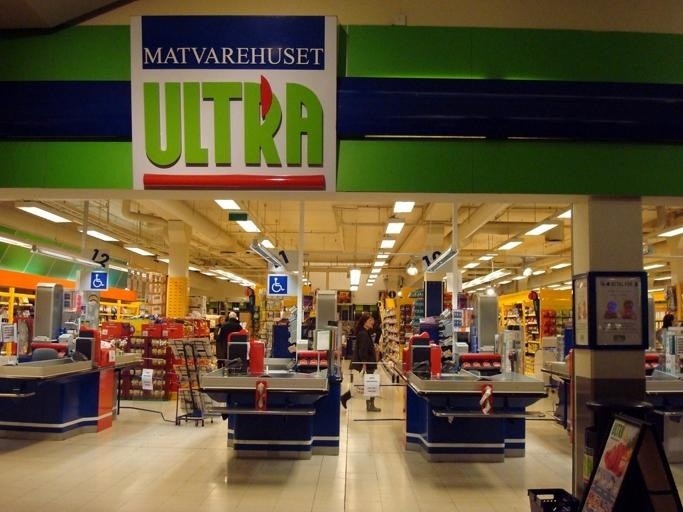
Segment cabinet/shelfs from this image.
[497,290,572,376]
[248,293,379,372]
[1,288,146,372]
[375,285,477,386]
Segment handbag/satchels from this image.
[349,368,372,401]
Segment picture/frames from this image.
[571,271,593,350]
[592,272,648,352]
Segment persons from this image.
[340,314,385,410]
[214,316,226,369]
[219,311,246,360]
[655,313,674,343]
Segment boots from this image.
[340,390,351,409]
[366,398,382,412]
